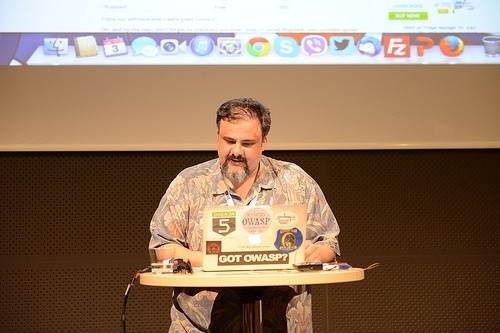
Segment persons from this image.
[148,97,340,333]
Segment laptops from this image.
[204,204,307,272]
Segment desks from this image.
[139,267,365,333]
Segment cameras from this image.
[292,262,323,272]
